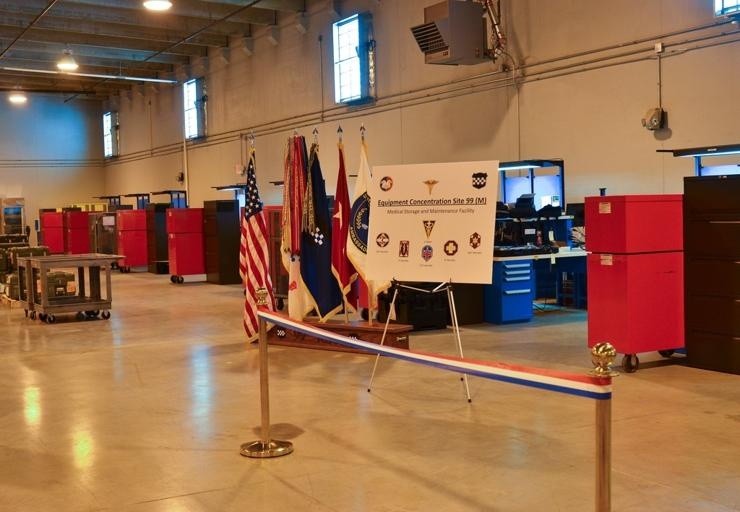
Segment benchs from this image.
[237,132,378,342]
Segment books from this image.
[0,247,77,301]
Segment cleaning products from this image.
[16,253,87,320]
[33,252,126,323]
[584,195,686,374]
[116,208,147,274]
[165,208,206,283]
[329,207,377,320]
[87,211,116,270]
[239,205,288,310]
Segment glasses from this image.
[143,3,174,11]
[7,81,29,108]
[56,39,81,74]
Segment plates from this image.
[683,175,740,376]
[37,199,290,309]
[584,193,686,356]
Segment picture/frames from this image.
[484,216,592,327]
